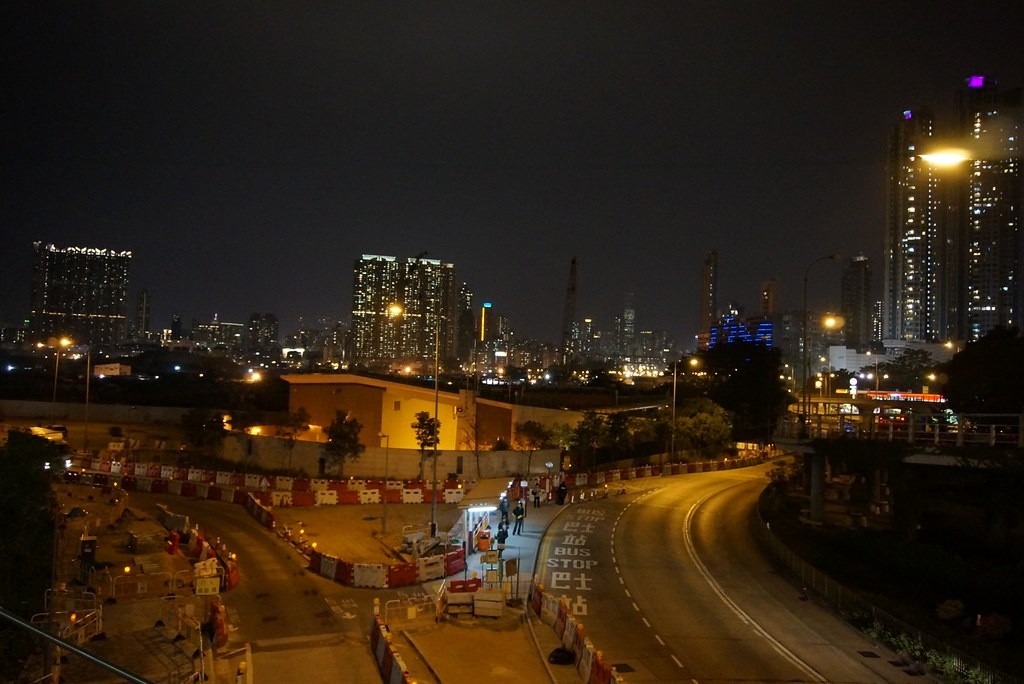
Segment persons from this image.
[495,482,566,561]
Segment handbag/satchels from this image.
[532,490,537,495]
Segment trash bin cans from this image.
[478,530,491,550]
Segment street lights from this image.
[481,302,492,342]
[37,342,60,403]
[671,357,699,467]
[60,336,91,454]
[390,304,437,537]
[797,252,843,439]
